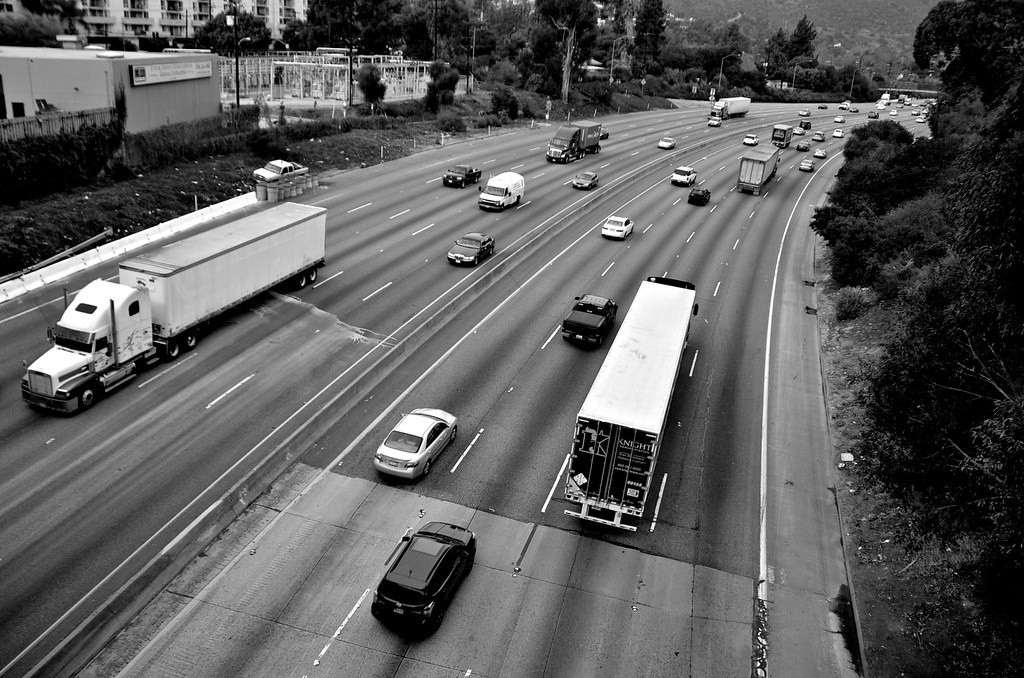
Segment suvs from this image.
[370,521,478,638]
[561,294,619,351]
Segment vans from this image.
[478,171,525,212]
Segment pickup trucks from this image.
[443,164,482,189]
[252,159,309,185]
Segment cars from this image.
[572,171,600,191]
[658,94,937,196]
[447,232,496,268]
[688,187,712,206]
[601,216,635,241]
[372,407,460,485]
[601,129,609,140]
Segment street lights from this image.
[850,67,870,96]
[718,54,741,89]
[609,35,636,87]
[234,6,252,107]
[793,59,813,88]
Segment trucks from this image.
[557,276,700,533]
[21,201,327,418]
[545,120,602,164]
